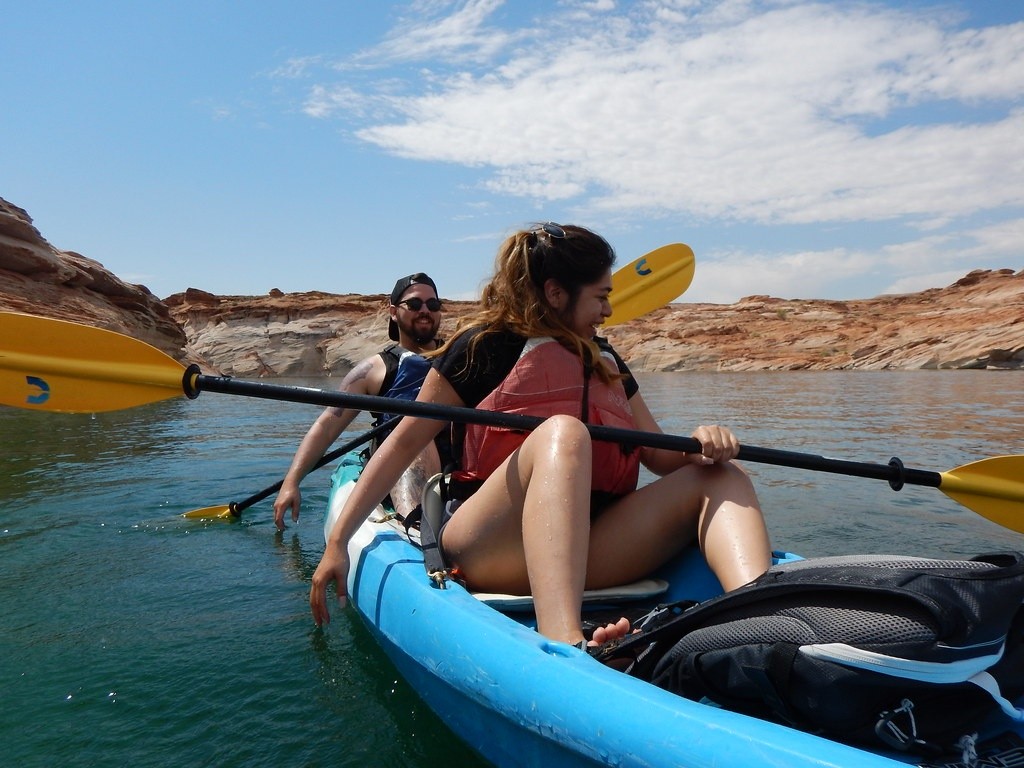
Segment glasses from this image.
[542,221,566,244]
[394,297,439,312]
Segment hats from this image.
[388,274,439,342]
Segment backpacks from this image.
[588,551,1024,767]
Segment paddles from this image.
[0,309,1023,534]
[184,241,697,523]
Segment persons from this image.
[310,223,772,669]
[274,274,448,532]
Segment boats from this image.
[322,448,920,767]
